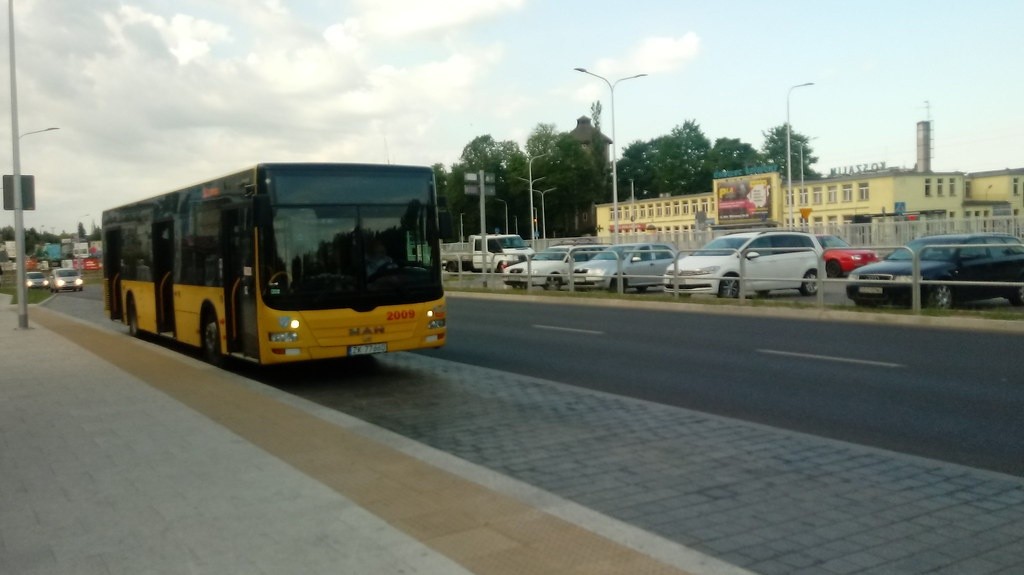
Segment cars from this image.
[573,243,686,293]
[49,268,84,294]
[27,271,50,290]
[846,233,1024,312]
[502,242,611,291]
[662,230,827,298]
[815,234,879,279]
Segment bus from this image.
[101,161,447,378]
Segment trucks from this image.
[440,233,536,273]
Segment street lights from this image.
[801,136,819,232]
[513,215,519,234]
[574,66,650,244]
[495,198,509,235]
[528,150,552,250]
[628,178,635,232]
[460,212,465,242]
[12,126,62,329]
[787,82,814,232]
[529,188,556,239]
